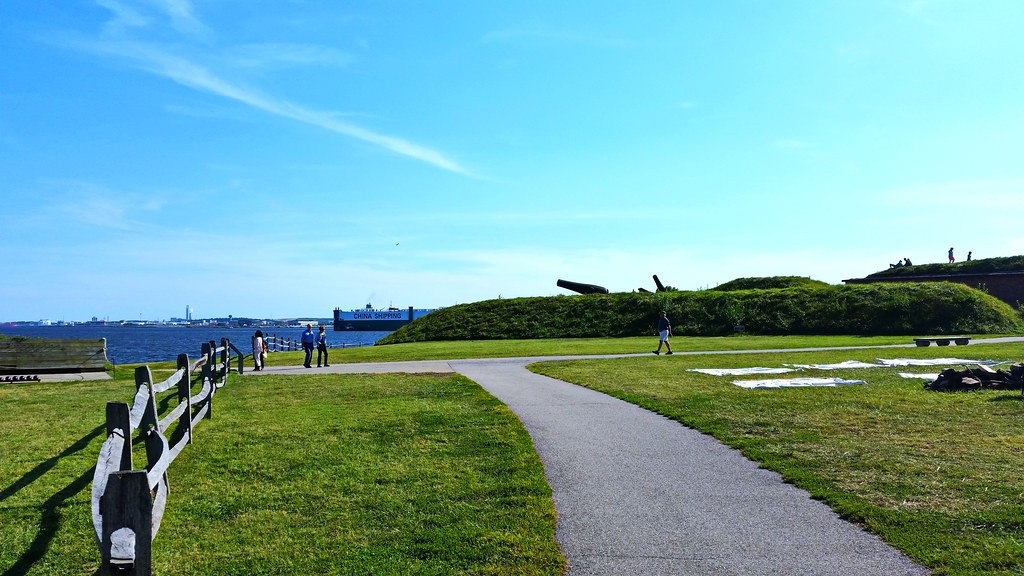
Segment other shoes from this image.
[308,366,311,368]
[303,364,308,368]
[665,351,673,355]
[324,364,330,367]
[317,365,322,367]
[652,351,659,355]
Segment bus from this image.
[389,307,399,312]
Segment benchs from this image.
[913,336,971,347]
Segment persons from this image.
[890,258,912,268]
[253,330,268,371]
[967,251,972,261]
[315,326,330,367]
[948,248,954,263]
[652,311,673,355]
[301,324,314,368]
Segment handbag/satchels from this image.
[262,351,268,359]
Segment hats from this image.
[319,326,326,329]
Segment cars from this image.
[138,322,144,325]
